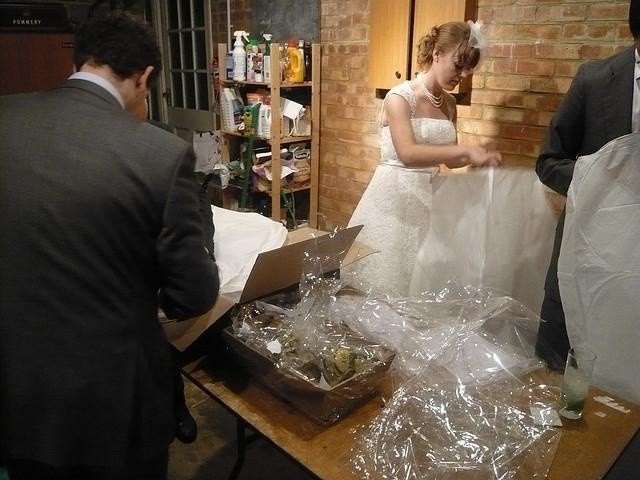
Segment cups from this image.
[558,346,597,419]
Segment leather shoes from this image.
[176,407,197,444]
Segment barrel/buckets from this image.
[284,39,304,83]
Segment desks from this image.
[178,285,640,480]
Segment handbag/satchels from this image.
[193,127,222,174]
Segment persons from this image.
[339,22,503,297]
[535,0,640,374]
[1,9,219,479]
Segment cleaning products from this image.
[263,33,272,83]
[232,31,250,82]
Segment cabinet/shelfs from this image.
[368,0,477,94]
[218,43,322,229]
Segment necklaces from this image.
[415,73,445,109]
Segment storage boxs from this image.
[160,225,378,353]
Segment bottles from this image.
[297,39,312,81]
[224,52,233,80]
